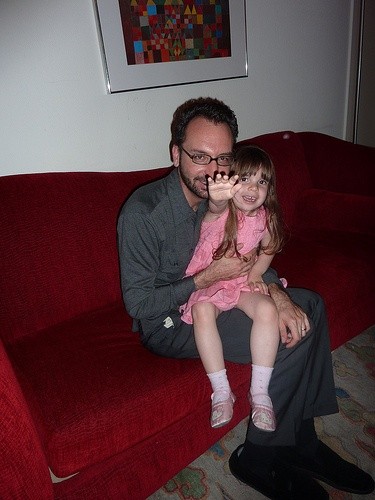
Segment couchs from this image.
[0,130,375,500]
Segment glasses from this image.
[181,145,235,166]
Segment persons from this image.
[177,143,281,434]
[116,95,375,499]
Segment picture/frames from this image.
[92,0,248,94]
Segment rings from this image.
[301,328,307,331]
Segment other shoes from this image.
[210,390,236,428]
[228,443,332,500]
[303,439,375,495]
[248,392,276,432]
